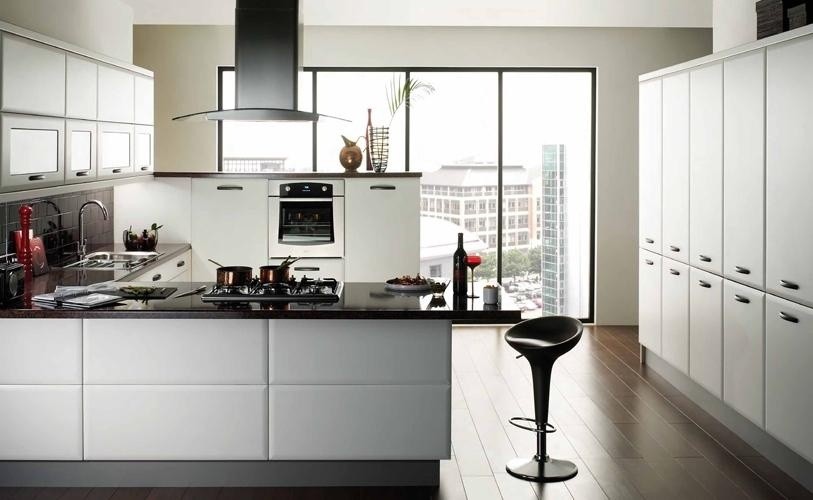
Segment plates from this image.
[383,278,433,290]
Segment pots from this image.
[206,258,252,286]
[259,258,302,284]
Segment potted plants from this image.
[367,69,437,173]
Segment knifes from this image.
[174,286,205,297]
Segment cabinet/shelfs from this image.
[0,30,64,195]
[689,265,724,403]
[638,69,662,255]
[127,259,169,284]
[134,70,154,177]
[722,38,767,292]
[269,257,344,283]
[764,33,813,308]
[661,66,688,266]
[636,249,662,356]
[96,58,134,181]
[189,175,267,281]
[169,249,190,284]
[725,276,765,430]
[766,294,811,463]
[64,48,97,185]
[662,257,690,377]
[688,58,722,277]
[344,176,420,284]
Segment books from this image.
[33,283,125,310]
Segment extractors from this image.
[170,0,352,125]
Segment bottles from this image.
[16,205,33,281]
[452,233,477,296]
[452,295,467,311]
[366,108,373,171]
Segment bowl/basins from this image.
[122,230,159,252]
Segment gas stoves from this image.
[201,274,343,303]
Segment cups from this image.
[483,286,498,304]
[426,296,450,311]
[425,279,450,297]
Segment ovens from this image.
[268,177,346,283]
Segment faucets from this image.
[77,200,109,252]
[27,198,63,249]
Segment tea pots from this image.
[338,134,369,171]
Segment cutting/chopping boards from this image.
[117,287,176,298]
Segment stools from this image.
[500,315,585,483]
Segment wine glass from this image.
[462,256,480,299]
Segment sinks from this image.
[59,249,160,272]
